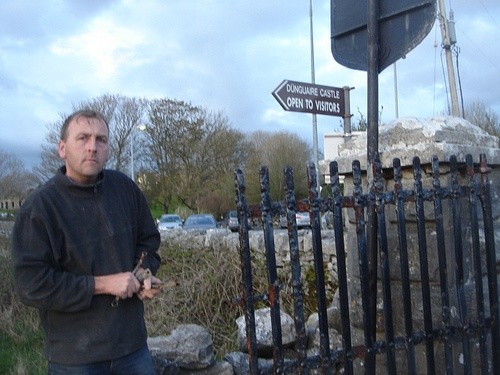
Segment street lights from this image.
[129,124,147,183]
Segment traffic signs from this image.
[271,78,346,118]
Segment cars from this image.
[279,209,311,230]
[224,210,255,232]
[158,214,183,231]
[183,213,223,233]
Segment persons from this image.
[10,108,162,375]
